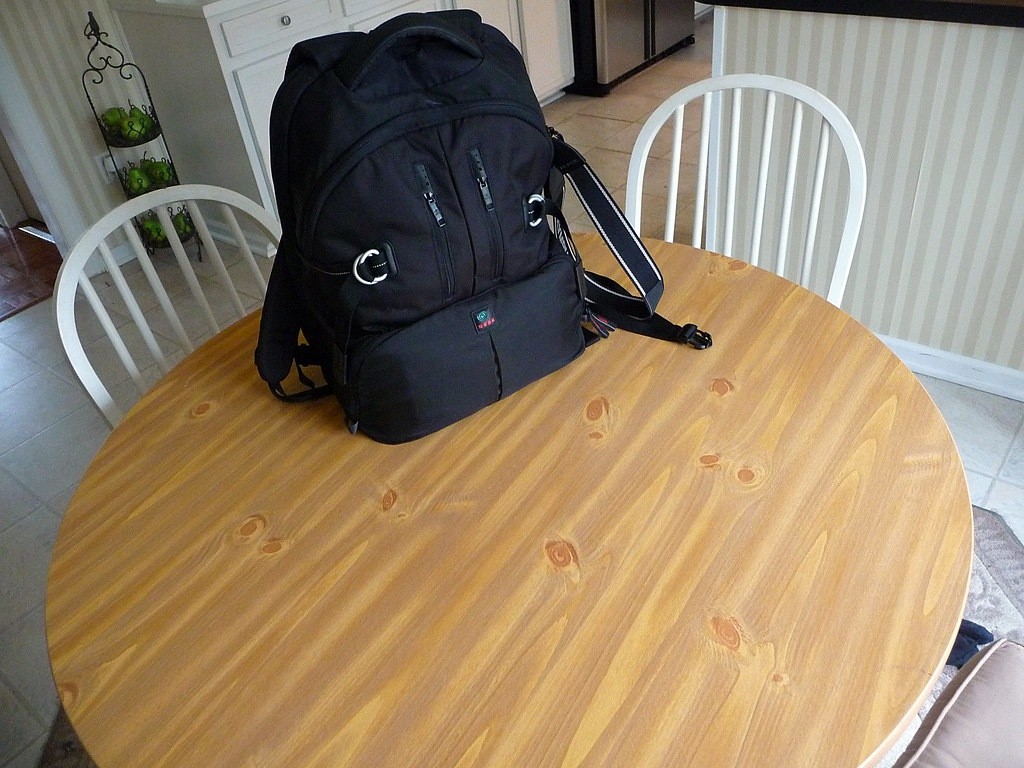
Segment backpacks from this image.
[256,10,713,446]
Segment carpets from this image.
[35,491,1024,768]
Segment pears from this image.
[127,150,173,195]
[100,97,154,142]
[140,210,190,243]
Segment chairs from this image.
[612,70,873,311]
[54,179,326,427]
[889,631,1024,768]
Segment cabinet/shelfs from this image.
[112,0,572,250]
[561,0,697,98]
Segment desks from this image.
[48,228,983,768]
